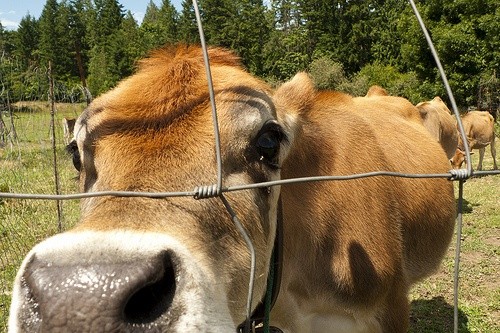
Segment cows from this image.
[414,95,459,161]
[447,110,498,171]
[6,41,457,333]
